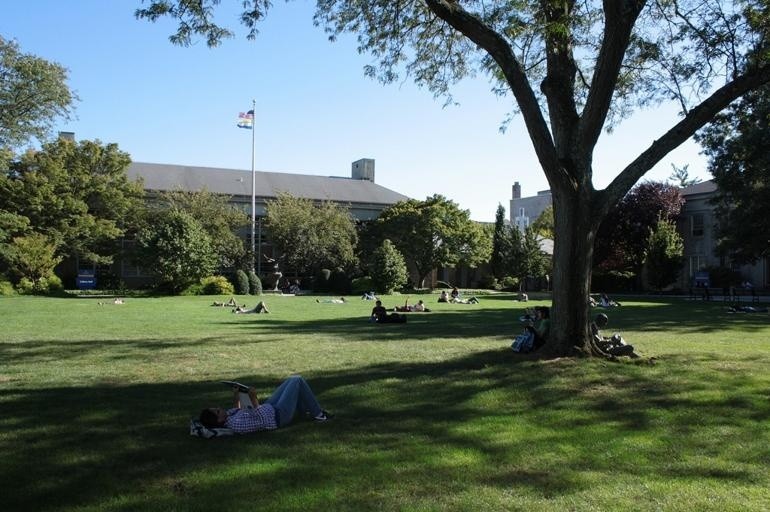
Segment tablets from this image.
[221,381,249,393]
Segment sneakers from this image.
[311,412,337,425]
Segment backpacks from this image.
[519,325,538,354]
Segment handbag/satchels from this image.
[188,418,236,440]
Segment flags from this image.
[237,109,253,129]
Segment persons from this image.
[523,306,552,346]
[732,304,770,314]
[198,376,337,438]
[702,278,711,301]
[315,297,349,305]
[96,296,127,306]
[589,294,597,307]
[211,296,246,308]
[588,312,641,360]
[232,301,270,315]
[362,286,480,323]
[741,279,757,295]
[599,290,621,307]
